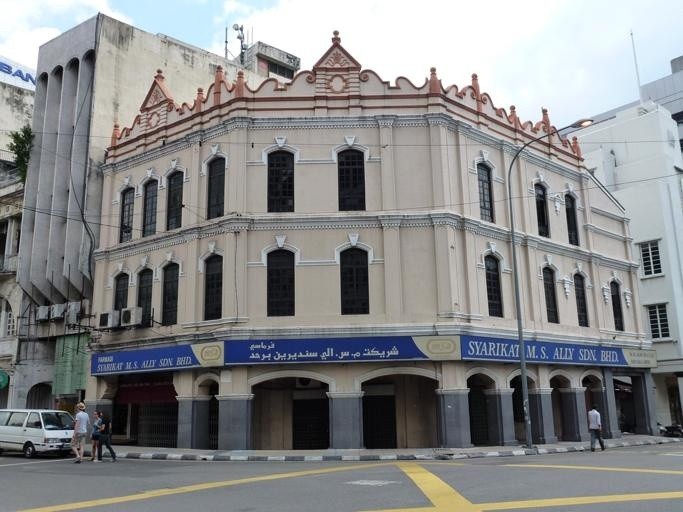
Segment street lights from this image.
[506,117,596,448]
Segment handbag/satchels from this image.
[91,432,105,441]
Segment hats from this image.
[77,402,85,409]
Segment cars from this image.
[664,424,683,437]
[657,421,668,435]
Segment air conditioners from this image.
[64,312,77,326]
[66,300,81,313]
[51,304,64,320]
[295,377,321,390]
[121,307,143,327]
[95,310,119,329]
[35,305,48,321]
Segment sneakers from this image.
[74,456,117,463]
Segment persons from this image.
[70,403,117,464]
[588,404,607,452]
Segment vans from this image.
[0,407,76,459]
